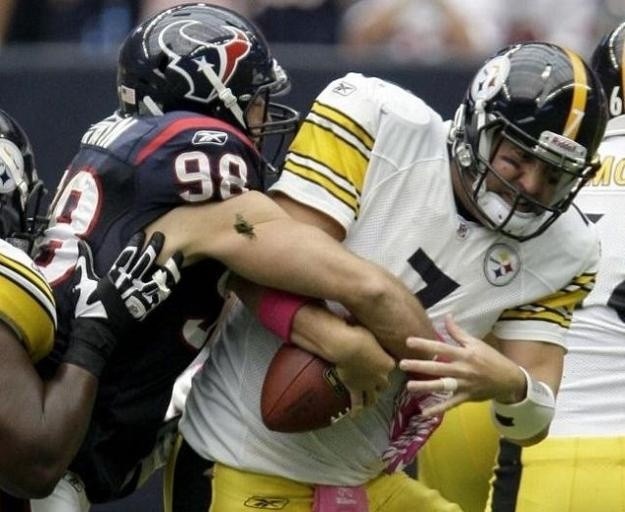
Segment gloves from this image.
[62,232,185,378]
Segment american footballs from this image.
[260,314,371,433]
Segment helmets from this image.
[0,110,48,240]
[117,3,298,177]
[447,23,625,241]
